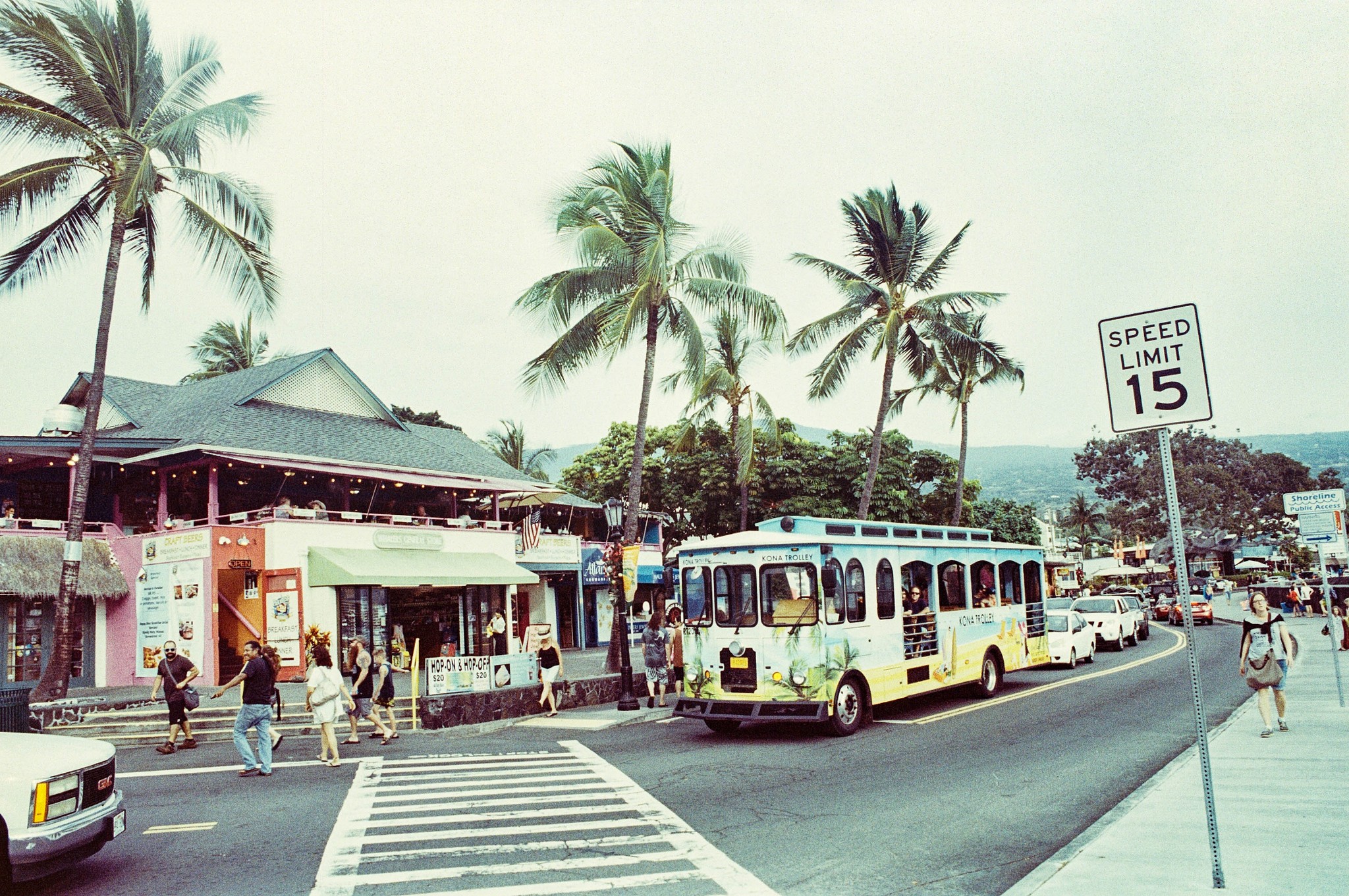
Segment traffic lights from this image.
[1075,568,1084,585]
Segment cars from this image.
[1187,576,1234,594]
[1265,576,1286,582]
[1298,570,1332,580]
[1151,597,1174,621]
[0,732,126,894]
[1123,595,1150,640]
[1168,594,1213,626]
[1045,610,1097,669]
[1044,596,1072,609]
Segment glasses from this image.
[911,592,920,595]
[164,648,177,652]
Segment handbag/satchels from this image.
[1245,648,1283,689]
[1285,599,1293,608]
[538,669,542,679]
[309,667,341,705]
[1320,622,1330,635]
[184,686,200,711]
[1330,590,1337,599]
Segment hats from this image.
[348,635,366,645]
[536,625,552,639]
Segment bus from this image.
[660,516,1048,736]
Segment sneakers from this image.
[178,740,197,748]
[156,743,174,753]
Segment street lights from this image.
[603,495,641,711]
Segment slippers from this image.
[326,763,341,766]
[380,731,396,745]
[340,739,360,744]
[658,703,668,707]
[647,696,654,708]
[546,712,557,717]
[369,733,384,738]
[317,754,328,761]
[535,700,542,712]
[382,735,399,738]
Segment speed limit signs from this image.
[1098,302,1212,434]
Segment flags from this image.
[522,507,540,551]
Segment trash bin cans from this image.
[0,686,33,733]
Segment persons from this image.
[339,635,397,745]
[368,648,400,738]
[909,587,930,658]
[640,612,671,709]
[962,583,1021,608]
[456,500,495,530]
[1238,592,1294,738]
[417,505,431,525]
[213,640,273,776]
[536,626,564,716]
[1044,572,1091,600]
[1206,583,1213,607]
[668,615,684,699]
[635,535,642,544]
[256,647,283,751]
[0,505,15,529]
[303,646,356,767]
[1223,580,1231,605]
[488,607,507,655]
[150,641,200,754]
[902,587,914,660]
[1246,565,1349,651]
[304,500,330,521]
[275,496,299,519]
[257,495,278,519]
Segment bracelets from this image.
[348,699,354,701]
[306,703,310,705]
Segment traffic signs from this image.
[1282,487,1346,534]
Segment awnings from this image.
[308,545,540,586]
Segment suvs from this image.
[1069,594,1139,651]
[1150,584,1176,610]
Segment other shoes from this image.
[257,771,271,776]
[272,735,284,751]
[239,768,259,776]
[1261,728,1274,737]
[1338,647,1348,651]
[1290,612,1327,618]
[1279,721,1289,732]
